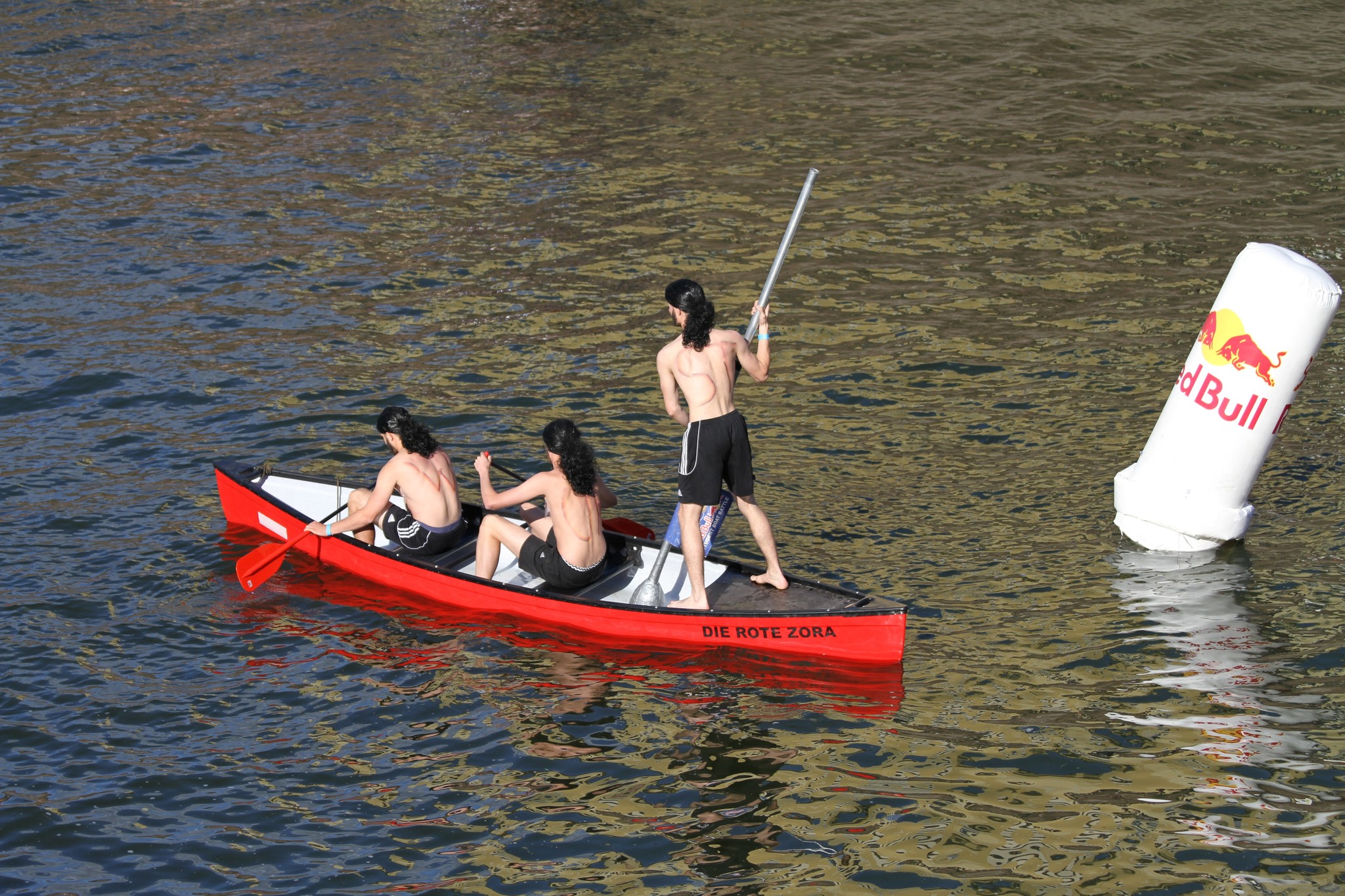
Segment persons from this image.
[473,418,618,588]
[303,406,467,555]
[656,279,789,609]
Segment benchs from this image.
[533,549,636,596]
[396,525,480,569]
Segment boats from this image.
[211,456,910,685]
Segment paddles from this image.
[233,499,353,596]
[474,449,656,540]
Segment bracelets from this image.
[757,334,769,340]
[326,523,332,536]
